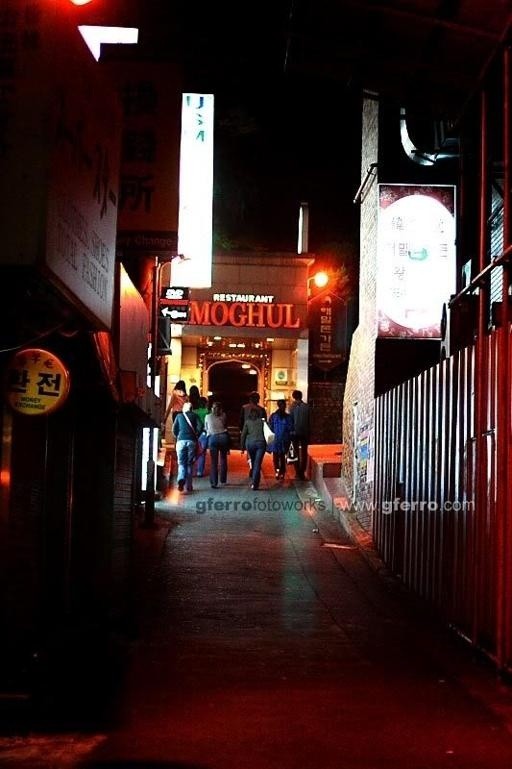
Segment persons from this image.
[165,380,229,493]
[240,407,268,490]
[287,389,312,483]
[239,393,268,476]
[267,400,295,480]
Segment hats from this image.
[182,402,193,413]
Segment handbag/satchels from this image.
[262,421,276,445]
[285,440,299,465]
[197,431,208,450]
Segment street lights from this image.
[147,252,191,526]
